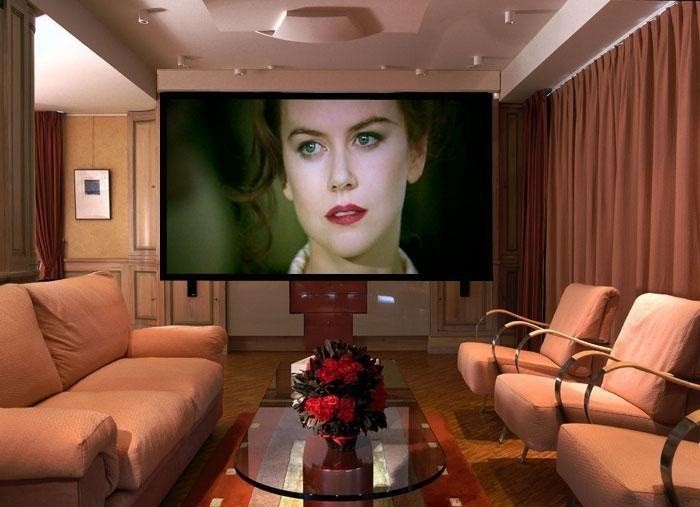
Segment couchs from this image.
[0,270,230,507]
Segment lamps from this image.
[474,56,482,67]
[235,70,247,75]
[177,56,187,67]
[416,68,423,76]
[137,7,148,25]
[505,12,516,24]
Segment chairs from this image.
[458,282,621,442]
[554,362,700,506]
[493,293,700,464]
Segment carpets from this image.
[181,414,497,507]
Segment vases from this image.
[326,432,356,450]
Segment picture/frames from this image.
[72,168,113,221]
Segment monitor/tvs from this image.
[158,88,494,284]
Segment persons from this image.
[221,98,440,275]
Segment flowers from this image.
[289,338,391,436]
